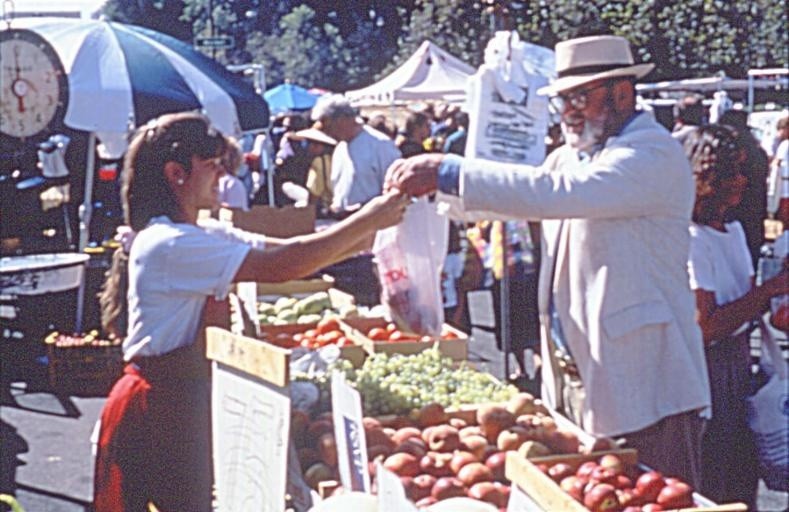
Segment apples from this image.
[295,401,694,512]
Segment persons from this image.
[214,100,541,383]
[382,35,712,491]
[88,111,413,512]
[37,130,81,248]
[674,109,789,511]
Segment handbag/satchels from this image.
[745,315,789,491]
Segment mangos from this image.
[256,292,358,325]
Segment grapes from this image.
[295,347,520,418]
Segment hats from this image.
[536,35,656,96]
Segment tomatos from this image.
[260,318,458,349]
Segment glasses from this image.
[548,82,607,114]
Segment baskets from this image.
[44,331,123,395]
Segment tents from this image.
[344,39,476,106]
